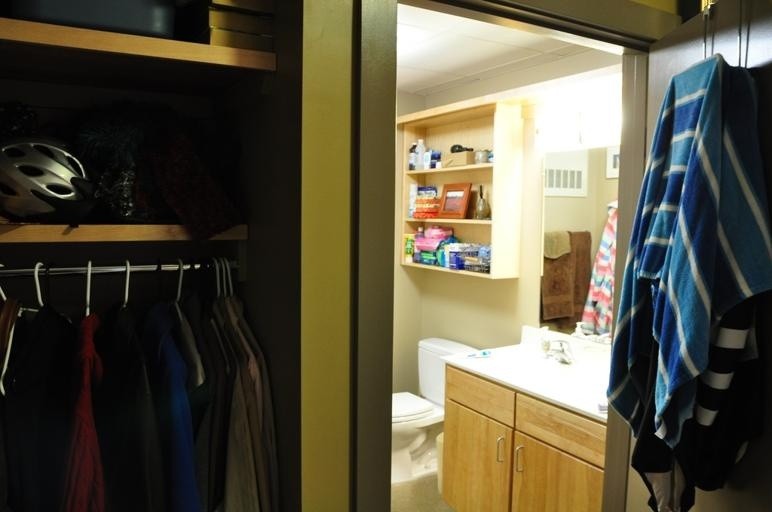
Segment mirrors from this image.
[539,144,623,346]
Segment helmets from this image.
[0,142,99,217]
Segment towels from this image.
[606,52,772,511]
[539,198,618,340]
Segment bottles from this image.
[408,139,425,170]
[476,198,490,219]
[450,144,474,153]
[413,226,424,263]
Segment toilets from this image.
[390,337,477,511]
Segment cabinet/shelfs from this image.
[397,98,524,281]
[439,326,614,512]
[1,0,355,512]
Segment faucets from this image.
[543,341,571,364]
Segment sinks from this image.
[440,326,611,422]
